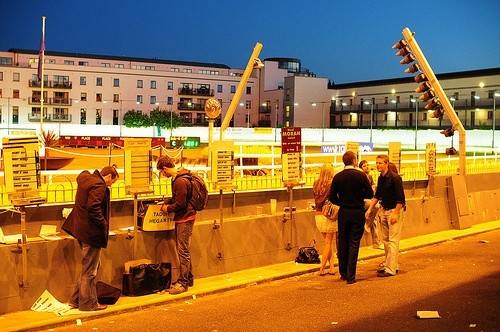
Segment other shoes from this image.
[319,270,337,276]
[364,222,370,233]
[171,280,193,286]
[377,243,384,249]
[340,274,356,283]
[169,282,188,293]
[97,303,108,308]
[377,265,398,276]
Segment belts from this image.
[383,207,391,210]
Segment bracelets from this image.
[393,213,400,218]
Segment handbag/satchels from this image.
[95,279,121,304]
[137,198,176,231]
[122,261,172,295]
[295,244,320,263]
[322,200,339,220]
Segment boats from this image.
[39,155,76,170]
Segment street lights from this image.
[364,101,373,143]
[450,98,455,148]
[492,93,500,155]
[410,98,418,150]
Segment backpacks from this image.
[177,169,209,210]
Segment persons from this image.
[156,153,198,294]
[366,155,405,277]
[359,159,385,250]
[330,151,374,284]
[386,163,407,212]
[61,163,119,311]
[313,162,337,275]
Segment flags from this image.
[37,26,46,83]
